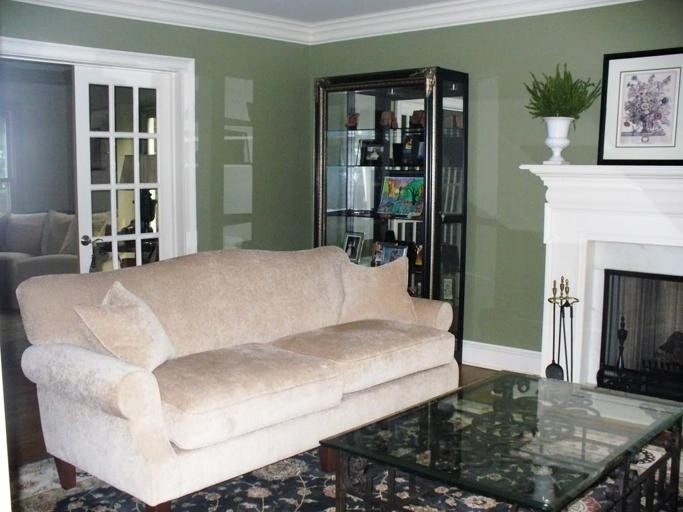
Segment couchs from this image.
[0,210,111,311]
[17,245,460,512]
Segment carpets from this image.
[11,398,683,512]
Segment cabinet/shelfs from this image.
[314,66,469,367]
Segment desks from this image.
[319,370,683,512]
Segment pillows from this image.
[72,281,177,373]
[0,211,111,254]
[335,256,418,325]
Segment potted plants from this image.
[521,62,601,165]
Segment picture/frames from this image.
[382,245,409,266]
[342,232,364,264]
[597,46,683,165]
[360,139,390,167]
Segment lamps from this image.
[119,154,157,244]
